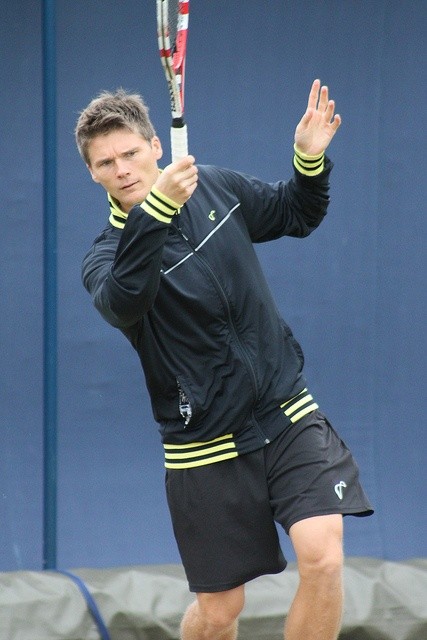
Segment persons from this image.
[72,77,374,640]
